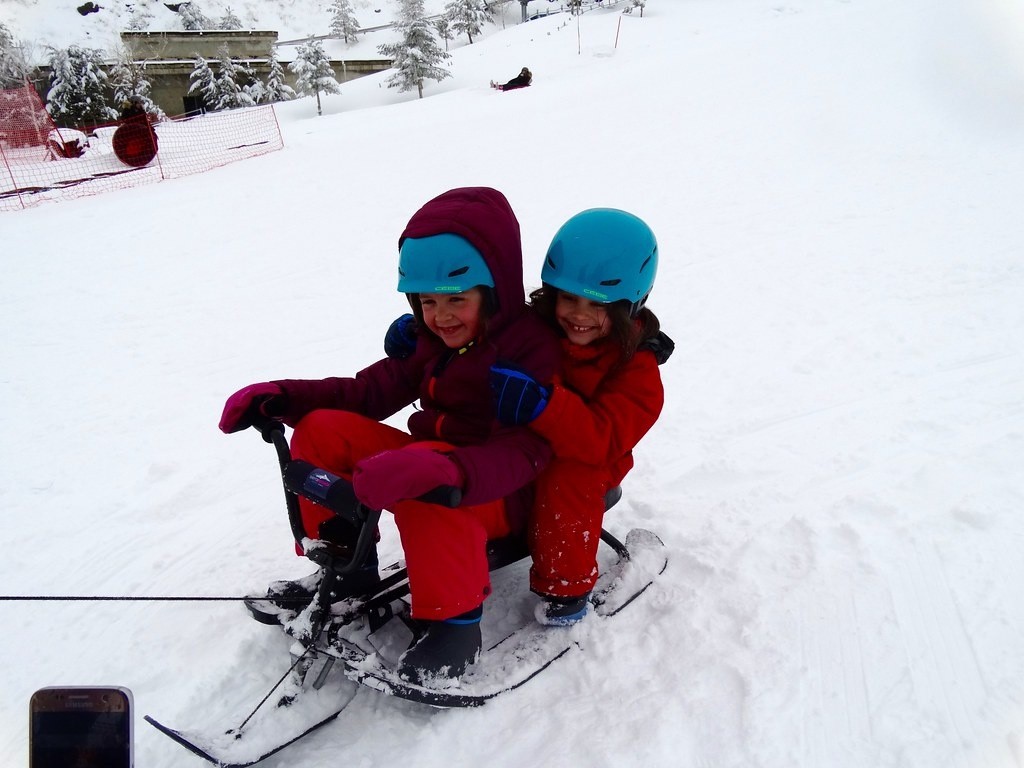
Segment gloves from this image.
[218,383,288,435]
[489,364,548,424]
[353,449,465,512]
[384,313,419,361]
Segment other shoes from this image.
[534,589,593,625]
[397,602,483,689]
[269,547,381,611]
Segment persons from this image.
[220,185,566,679]
[118,97,156,134]
[490,67,533,91]
[385,206,677,625]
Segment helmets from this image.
[541,208,659,304]
[395,233,494,293]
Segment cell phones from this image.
[27,686,135,768]
